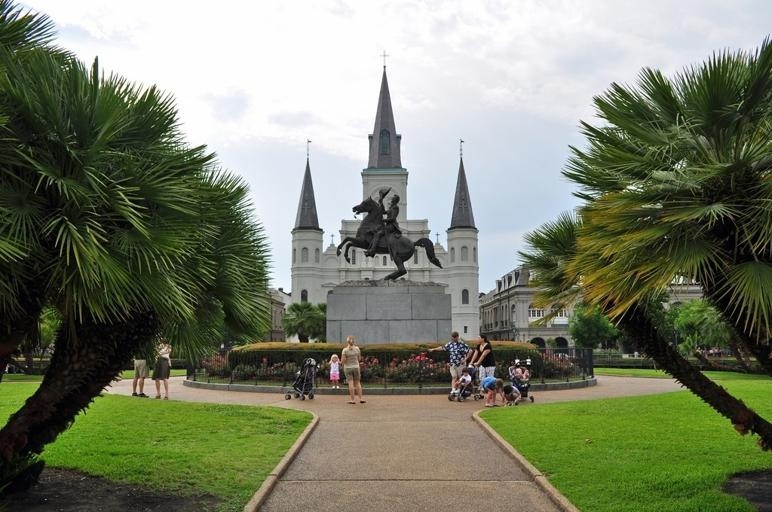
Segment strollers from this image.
[447,363,480,403]
[508,358,535,403]
[285,356,317,401]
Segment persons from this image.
[469,335,496,398]
[150,332,172,401]
[363,185,400,261]
[496,384,525,406]
[326,354,342,389]
[425,330,475,395]
[339,335,368,404]
[131,349,150,398]
[511,363,531,380]
[477,376,505,406]
[451,368,472,400]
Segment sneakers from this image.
[332,385,340,389]
[359,400,368,403]
[347,401,356,404]
[132,392,169,401]
[484,402,501,408]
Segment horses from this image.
[335,195,444,280]
